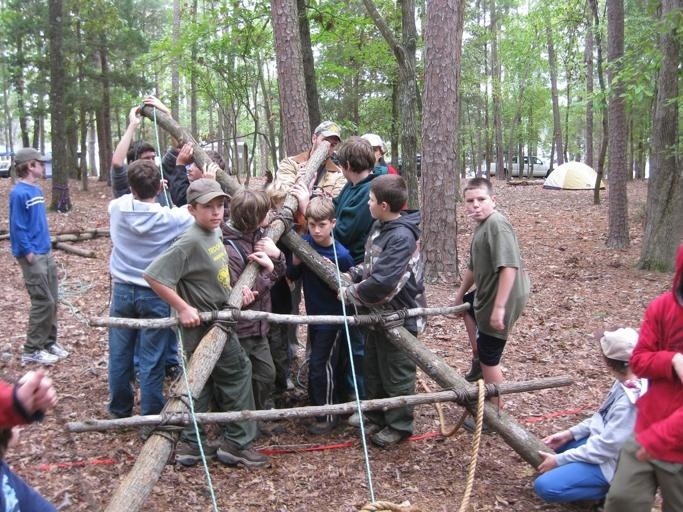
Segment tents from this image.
[541,160,606,191]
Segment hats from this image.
[359,133,388,154]
[314,120,342,142]
[185,178,232,205]
[15,147,52,164]
[600,327,639,362]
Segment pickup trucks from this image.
[475,155,554,177]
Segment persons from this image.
[604,243,682,511]
[102,92,430,470]
[532,325,649,504]
[7,146,70,365]
[451,177,530,435]
[0,428,59,512]
[0,366,59,431]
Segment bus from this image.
[200,142,246,177]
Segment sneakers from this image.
[463,419,490,433]
[465,357,482,381]
[174,423,282,466]
[306,411,407,447]
[21,344,70,365]
[135,365,183,384]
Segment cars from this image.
[383,154,421,176]
[0,159,15,178]
